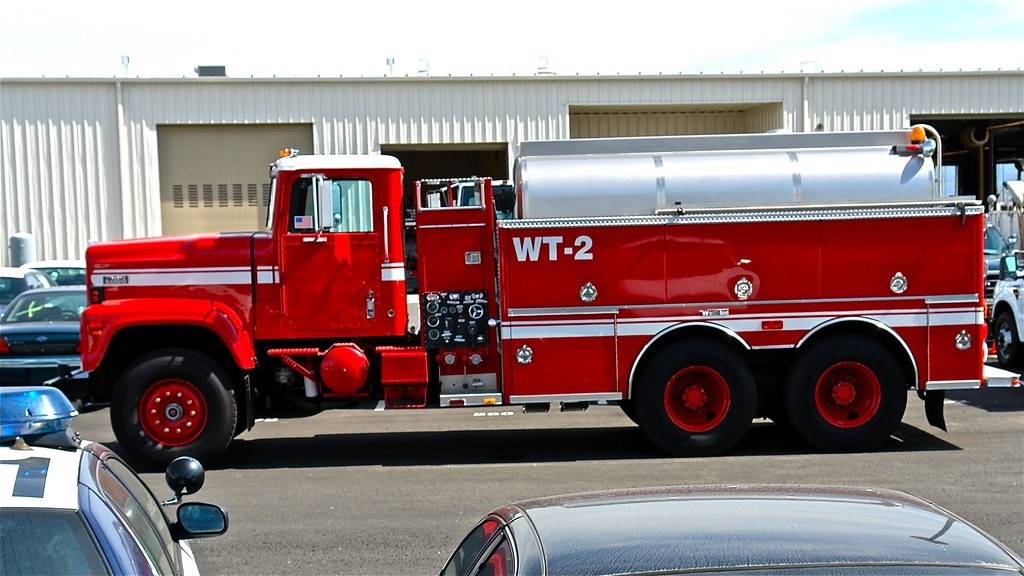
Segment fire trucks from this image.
[77,124,1019,474]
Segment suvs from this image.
[981,222,1024,369]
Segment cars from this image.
[0,267,58,323]
[0,385,229,576]
[0,285,88,387]
[437,483,1024,576]
[18,259,87,286]
